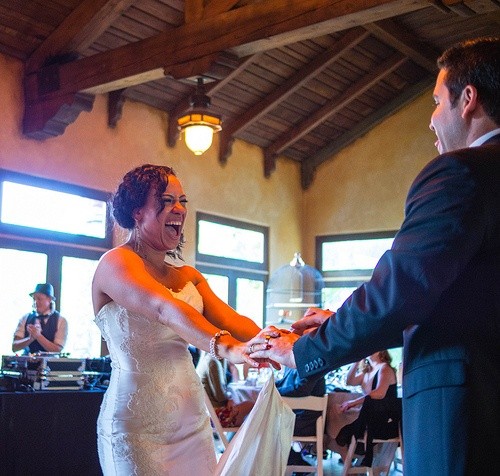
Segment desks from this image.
[0,389,107,476]
[228,381,364,419]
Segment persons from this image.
[188,342,258,455]
[248,36,500,476]
[12,283,66,356]
[335,349,400,476]
[273,365,327,476]
[91,164,336,476]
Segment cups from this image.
[248,368,257,389]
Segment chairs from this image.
[203,387,239,448]
[343,398,402,476]
[280,394,329,476]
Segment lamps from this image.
[176,77,222,155]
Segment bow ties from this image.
[35,314,50,320]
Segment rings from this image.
[265,335,270,343]
[249,343,254,353]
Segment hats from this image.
[29,282,56,301]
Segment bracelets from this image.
[210,329,232,360]
